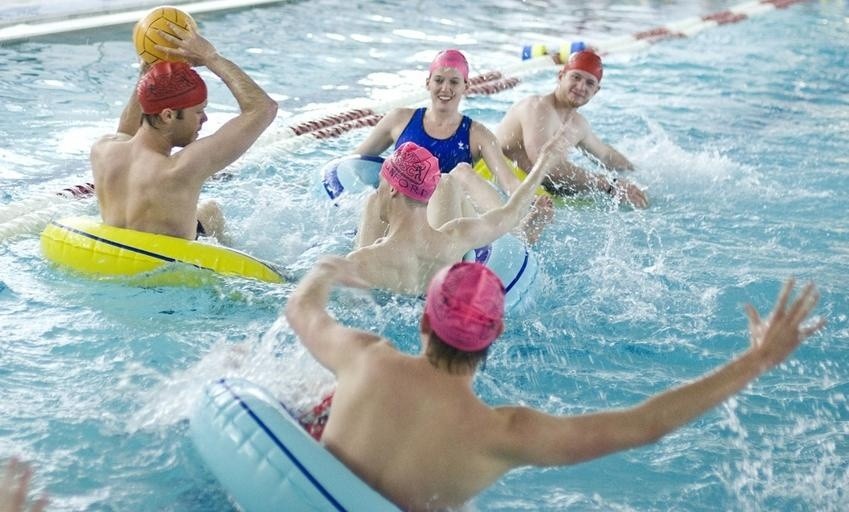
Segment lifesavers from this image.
[477,156,605,210]
[309,154,545,312]
[188,379,402,511]
[41,213,283,295]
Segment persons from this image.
[343,123,569,297]
[491,48,651,210]
[351,48,557,249]
[281,254,828,512]
[88,16,280,247]
[1,452,51,512]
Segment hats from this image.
[380,141,440,202]
[564,50,602,82]
[427,262,505,352]
[137,62,207,114]
[429,50,468,82]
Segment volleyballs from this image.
[134,6,198,67]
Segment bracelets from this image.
[607,178,617,193]
